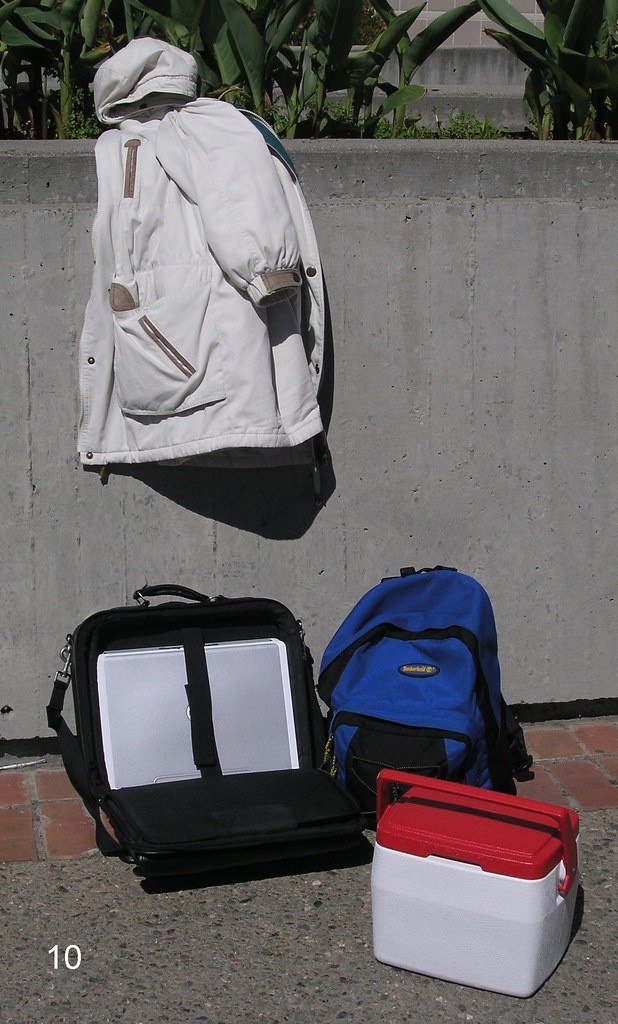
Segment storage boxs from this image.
[370,767,581,997]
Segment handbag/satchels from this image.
[47,584,367,878]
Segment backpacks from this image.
[317,564,533,815]
